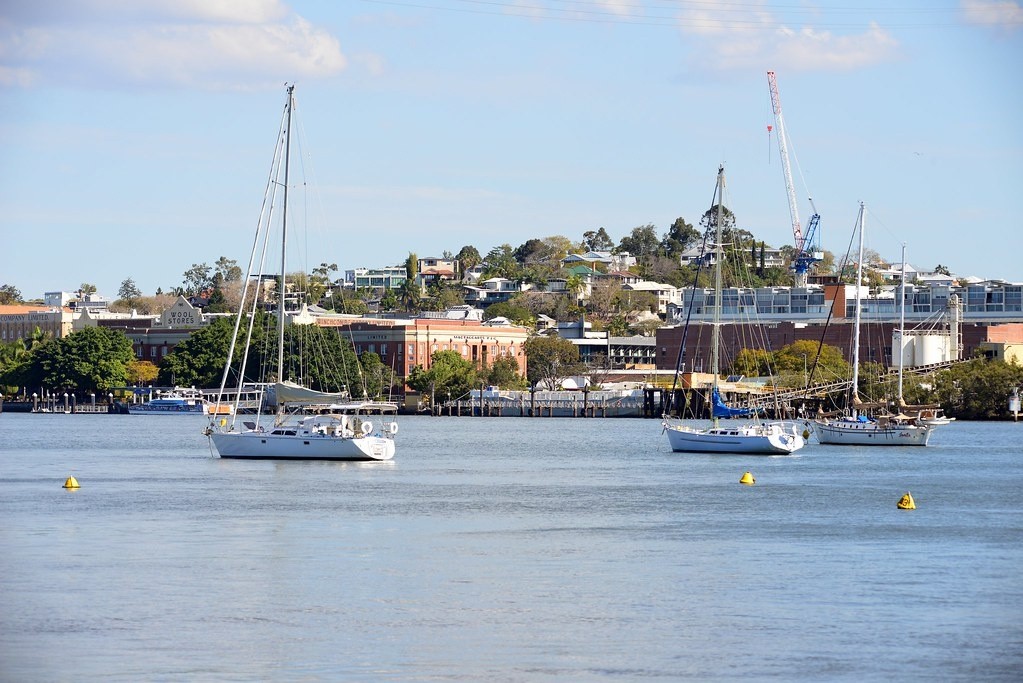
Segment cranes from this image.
[764,70,825,288]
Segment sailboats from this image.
[814,201,956,447]
[202,81,400,461]
[662,163,816,453]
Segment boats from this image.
[127,384,215,416]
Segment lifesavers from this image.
[580,408,586,415]
[360,421,373,434]
[389,422,399,434]
[527,408,534,416]
